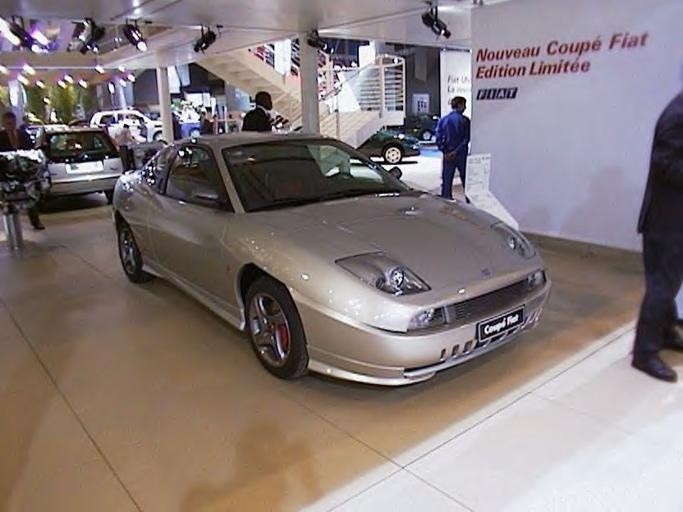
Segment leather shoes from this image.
[34,221,44,228]
[633,355,676,381]
[666,323,683,348]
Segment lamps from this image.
[307,28,334,54]
[422,1,450,39]
[9,17,216,56]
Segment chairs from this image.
[265,144,320,203]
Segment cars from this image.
[24,104,225,151]
[352,130,420,166]
[32,125,123,204]
[111,129,552,388]
[394,113,442,142]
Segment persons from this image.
[435,96,470,204]
[318,60,362,114]
[19,114,30,128]
[241,92,272,131]
[138,117,147,138]
[172,104,238,141]
[115,124,138,173]
[629,90,683,382]
[0,112,46,231]
